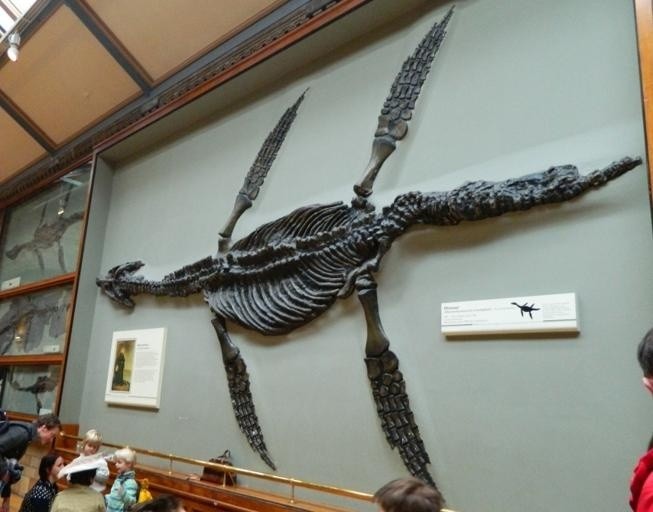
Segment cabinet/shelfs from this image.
[0,155,114,425]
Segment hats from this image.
[57,453,100,479]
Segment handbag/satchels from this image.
[201,450,236,486]
[135,478,152,504]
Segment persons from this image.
[50,467,105,512]
[20,453,65,512]
[0,411,62,512]
[57,428,109,492]
[369,477,445,512]
[630,325,652,512]
[106,448,138,511]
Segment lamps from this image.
[6,32,22,62]
